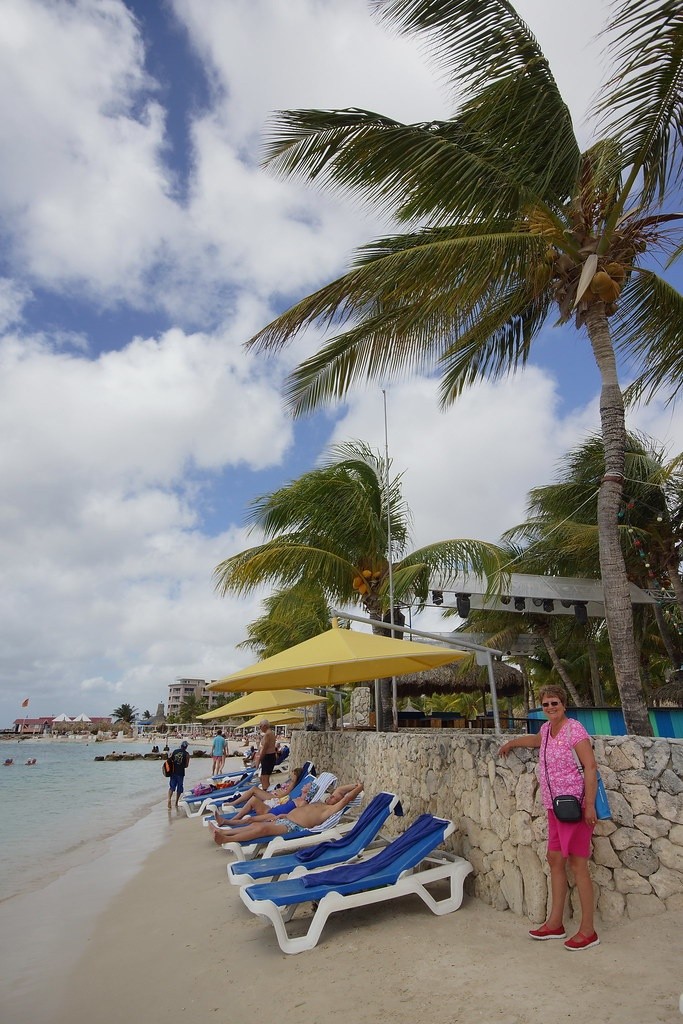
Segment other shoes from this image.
[564,929,600,951]
[528,923,567,939]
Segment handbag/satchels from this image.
[588,770,612,820]
[553,795,582,820]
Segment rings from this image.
[591,821,595,824]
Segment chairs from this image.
[177,747,474,955]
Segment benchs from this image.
[478,716,549,734]
[389,710,510,728]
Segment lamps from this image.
[432,590,444,606]
[455,593,472,617]
[501,596,588,625]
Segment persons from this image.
[151,720,364,845]
[497,685,600,951]
[4,758,35,765]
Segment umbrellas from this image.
[196,622,471,730]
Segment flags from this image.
[22,699,28,707]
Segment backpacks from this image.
[162,757,175,777]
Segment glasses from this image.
[542,701,561,707]
